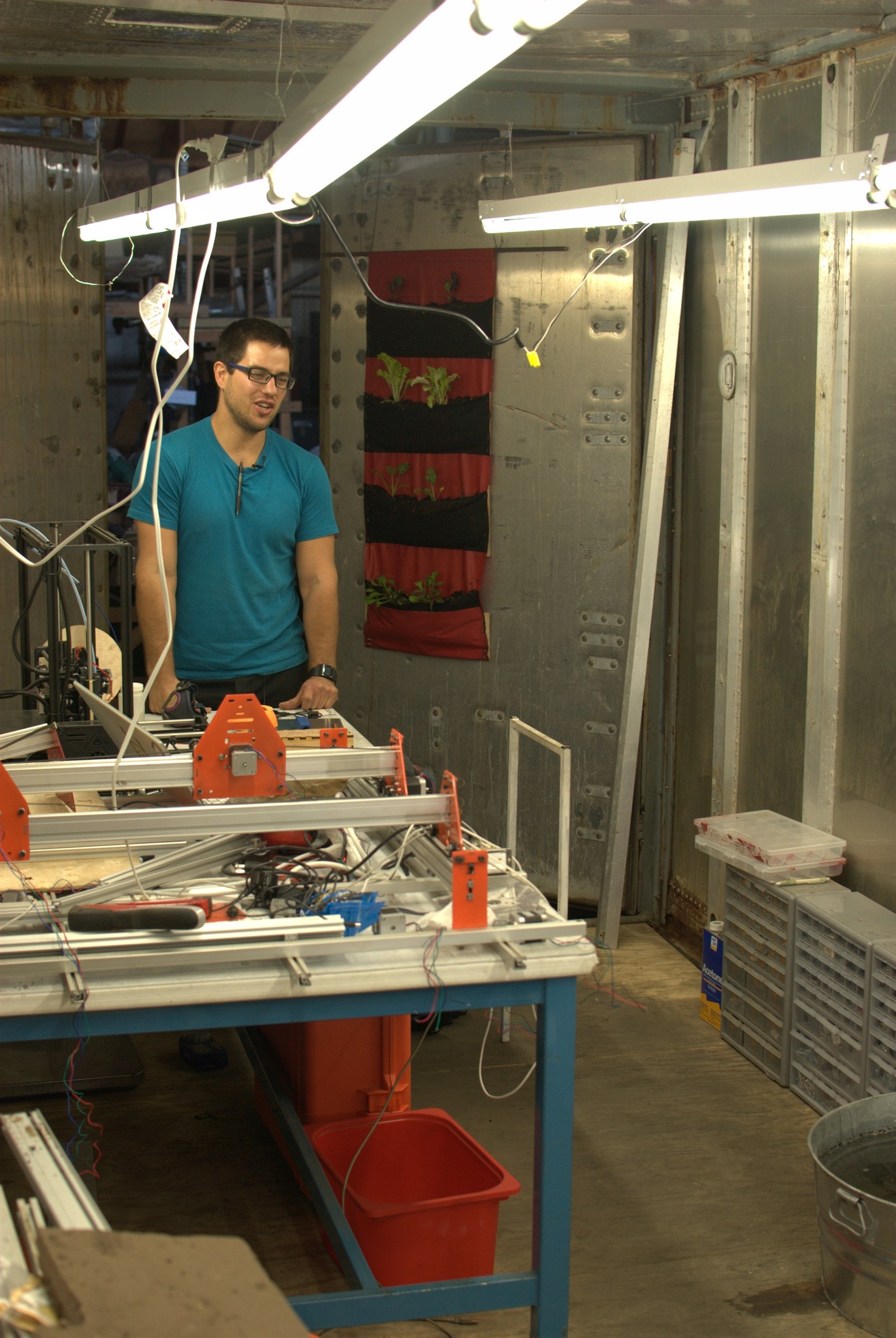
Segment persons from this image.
[125,318,338,713]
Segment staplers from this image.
[68,897,211,931]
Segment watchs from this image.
[307,664,337,686]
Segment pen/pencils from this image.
[235,462,243,517]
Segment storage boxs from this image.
[692,808,896,1120]
[303,1104,524,1289]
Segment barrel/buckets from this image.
[808,1091,895,1338]
[700,921,724,1032]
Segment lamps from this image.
[72,0,896,250]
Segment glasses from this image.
[226,363,296,390]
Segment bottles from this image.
[133,682,144,723]
